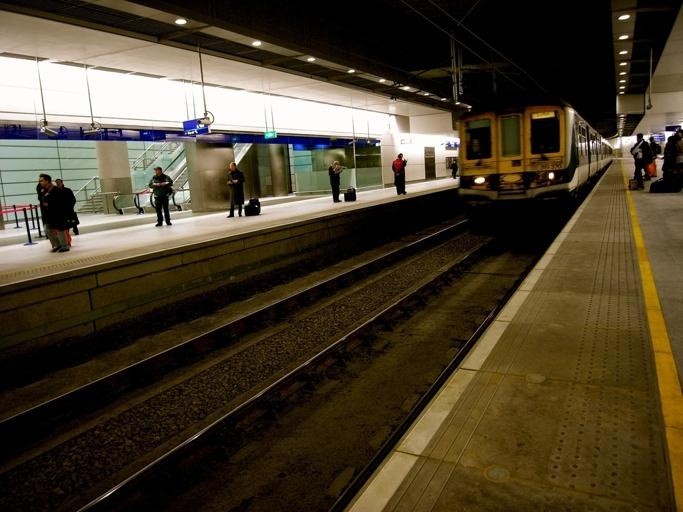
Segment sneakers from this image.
[51,246,70,252]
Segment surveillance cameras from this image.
[197,119,211,124]
[41,127,58,136]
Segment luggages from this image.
[244,192,260,216]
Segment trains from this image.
[455,97,614,211]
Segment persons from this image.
[392,152,407,195]
[662,128,683,181]
[149,166,173,226]
[630,133,657,190]
[328,161,343,202]
[227,162,246,218]
[449,160,458,180]
[36,174,79,253]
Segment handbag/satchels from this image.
[63,213,79,229]
[344,186,356,201]
[629,177,639,189]
